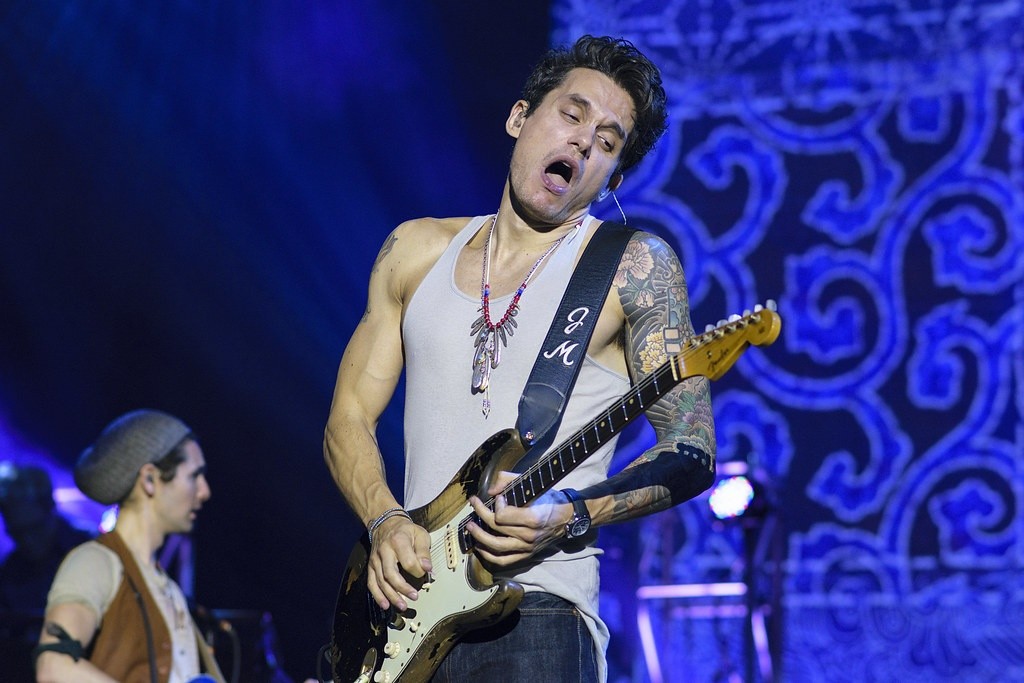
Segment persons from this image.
[0,467,300,683]
[32,407,230,683]
[320,34,717,681]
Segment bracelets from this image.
[366,508,413,545]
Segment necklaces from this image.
[471,206,583,419]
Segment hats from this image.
[71,408,190,506]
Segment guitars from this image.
[329,299,782,683]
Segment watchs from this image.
[560,487,592,539]
